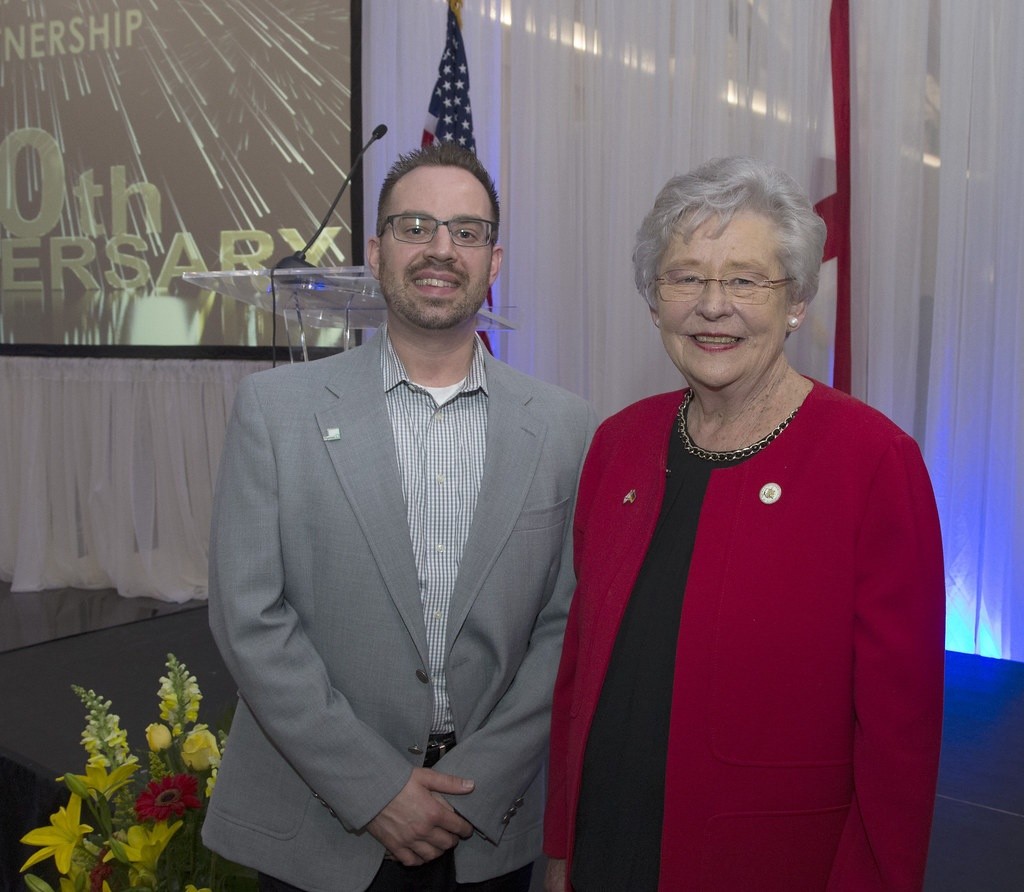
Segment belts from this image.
[423,736,457,770]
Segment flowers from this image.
[15,652,234,892]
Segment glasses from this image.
[652,268,796,304]
[378,213,499,248]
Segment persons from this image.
[200,155,595,891]
[540,153,945,892]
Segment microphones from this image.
[276,124,388,292]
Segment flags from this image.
[419,1,493,358]
